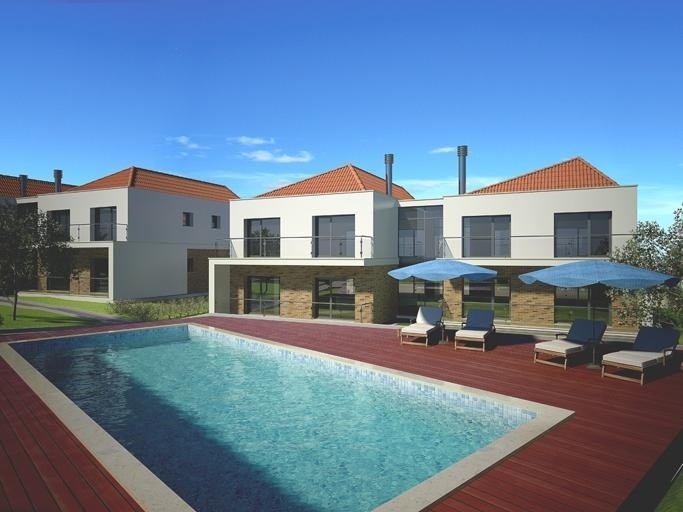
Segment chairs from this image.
[533,320,606,370]
[455,308,496,353]
[400,306,443,347]
[601,327,681,385]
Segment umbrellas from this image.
[386,258,499,339]
[515,259,679,364]
